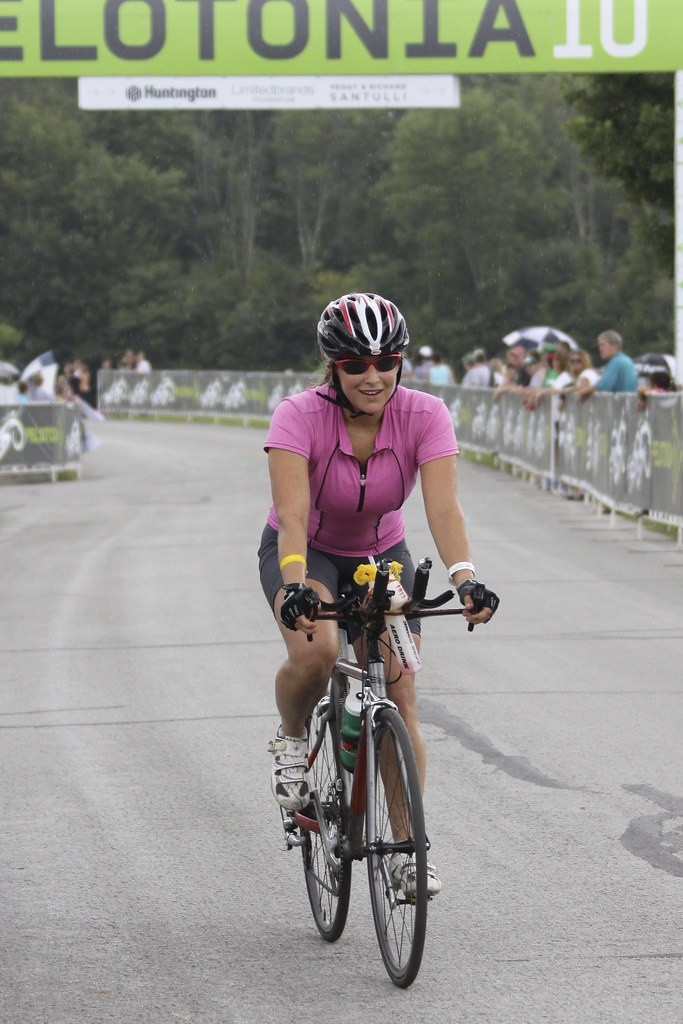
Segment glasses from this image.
[335,354,402,375]
[571,359,582,363]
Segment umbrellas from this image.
[0,360,20,380]
[493,325,580,354]
[18,349,61,404]
[629,351,678,384]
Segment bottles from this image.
[340,690,365,774]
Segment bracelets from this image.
[279,554,308,576]
[446,561,477,585]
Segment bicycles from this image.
[280,559,484,989]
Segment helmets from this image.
[318,293,410,365]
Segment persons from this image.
[16,347,152,404]
[397,326,678,412]
[256,292,499,896]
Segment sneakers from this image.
[267,723,311,810]
[388,852,441,898]
